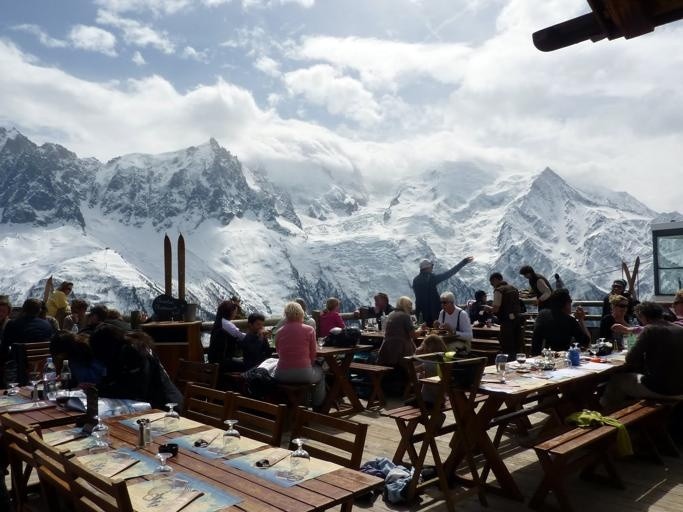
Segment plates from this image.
[519,295,537,302]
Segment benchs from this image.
[379,389,490,472]
[223,369,317,405]
[337,360,395,411]
[470,345,500,363]
[527,397,671,511]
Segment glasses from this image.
[432,264,434,266]
[674,301,681,304]
[618,303,628,308]
[440,301,449,304]
[68,288,71,291]
[612,285,622,288]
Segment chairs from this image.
[23,426,132,511]
[12,339,55,387]
[226,391,287,448]
[288,405,370,473]
[179,380,232,430]
[173,356,220,406]
[0,410,43,512]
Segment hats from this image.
[614,279,627,287]
[420,259,432,269]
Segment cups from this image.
[543,351,572,368]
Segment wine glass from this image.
[516,353,526,369]
[152,453,173,493]
[290,437,310,475]
[599,338,606,347]
[164,402,180,429]
[222,420,240,452]
[534,358,546,375]
[572,342,582,356]
[590,344,599,357]
[497,353,509,383]
[89,431,109,467]
[7,382,23,408]
[317,337,325,351]
[91,415,110,444]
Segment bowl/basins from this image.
[519,292,529,298]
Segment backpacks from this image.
[498,288,522,324]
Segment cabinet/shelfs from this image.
[140,319,203,384]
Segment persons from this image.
[434,292,474,355]
[0,280,183,413]
[533,288,592,354]
[318,298,345,339]
[555,273,562,288]
[413,258,474,327]
[245,315,270,371]
[601,280,683,417]
[209,301,246,383]
[377,296,426,397]
[356,292,395,330]
[404,334,448,429]
[274,299,325,412]
[518,266,555,312]
[471,290,495,327]
[482,272,527,357]
